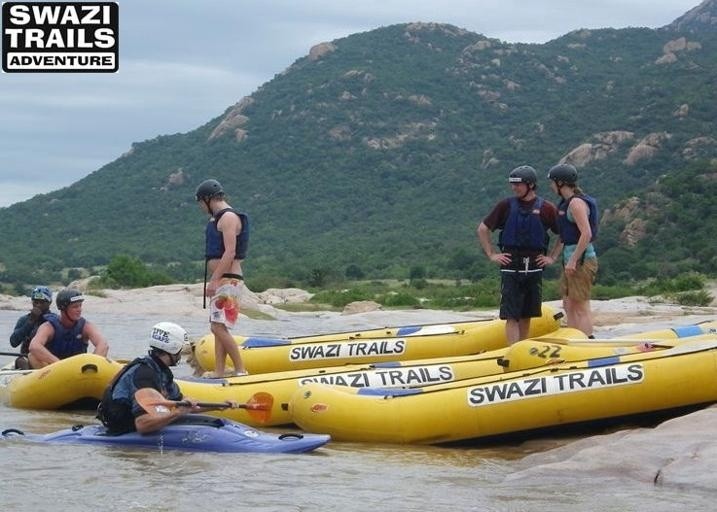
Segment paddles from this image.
[145,392,272,421]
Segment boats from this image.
[174,324,589,428]
[3,327,594,409]
[1,350,120,411]
[195,305,562,367]
[192,310,560,375]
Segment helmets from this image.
[547,163,580,182]
[509,164,539,184]
[151,321,192,357]
[57,289,85,309]
[31,286,54,304]
[194,179,224,200]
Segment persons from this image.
[29,290,107,369]
[195,179,249,377]
[547,164,600,338]
[10,288,54,369]
[477,164,558,348]
[98,324,239,431]
